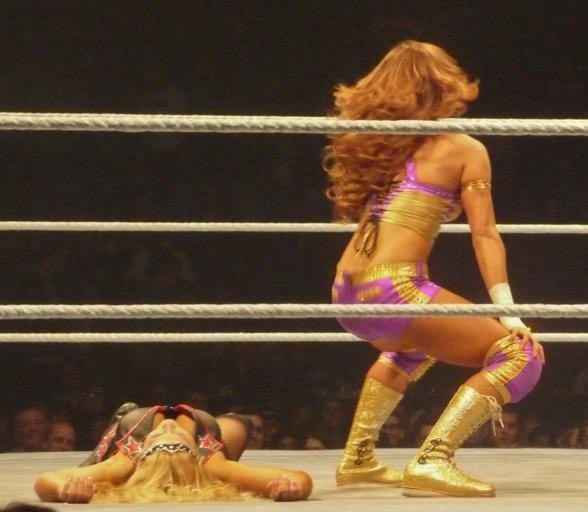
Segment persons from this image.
[0,342,588,452]
[32,398,315,506]
[322,38,547,502]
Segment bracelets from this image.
[486,283,529,332]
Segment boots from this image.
[404,385,505,499]
[338,379,403,486]
